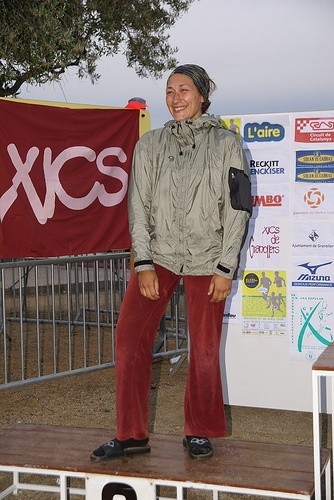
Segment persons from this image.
[90,64,251,462]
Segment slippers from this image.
[183,435,214,459]
[89,439,150,462]
